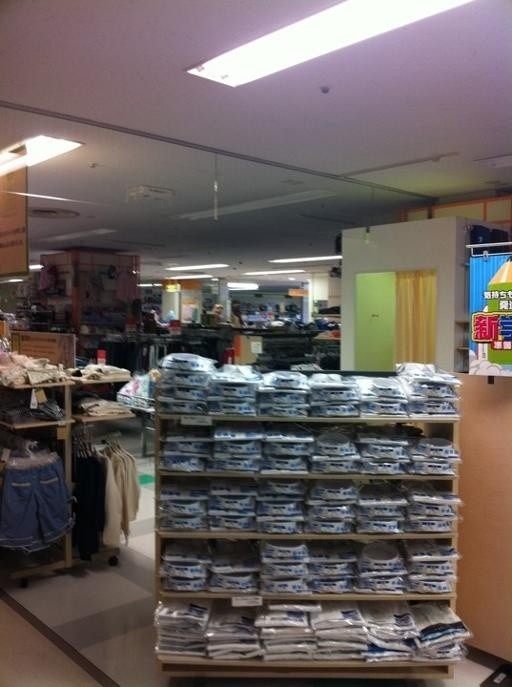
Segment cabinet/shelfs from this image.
[152,378,460,681]
[1,330,135,582]
[453,371,512,662]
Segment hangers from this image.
[15,436,38,460]
[72,437,136,463]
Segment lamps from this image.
[364,188,370,244]
